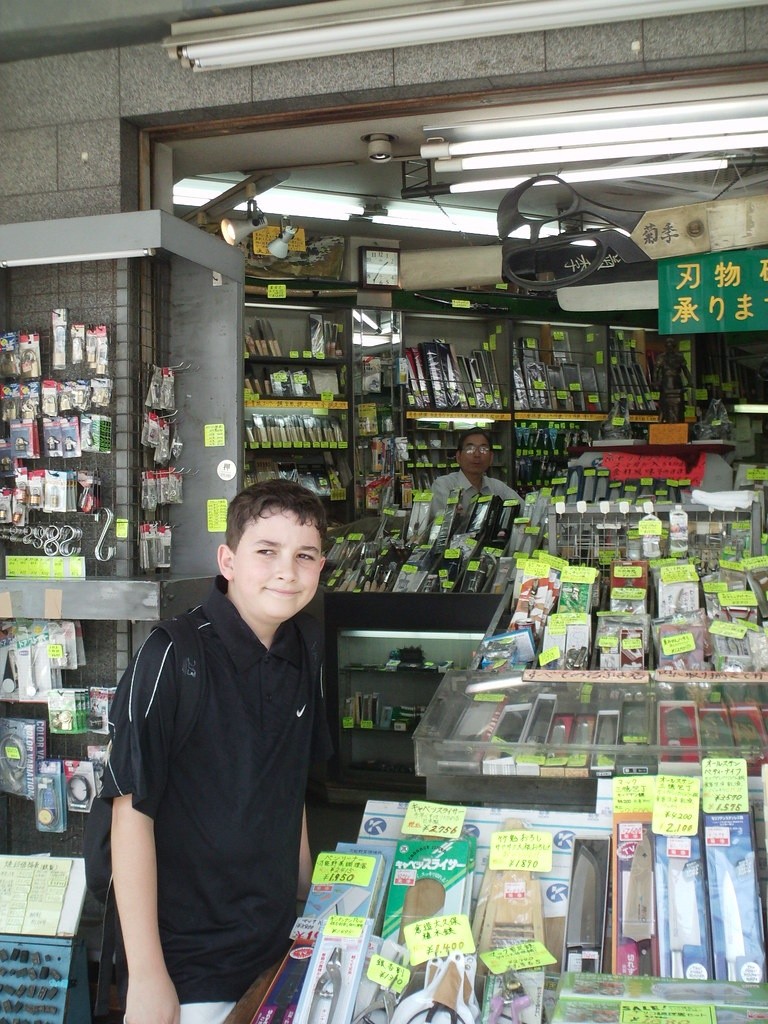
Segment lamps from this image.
[221,183,268,246]
[265,215,299,259]
[160,0,768,74]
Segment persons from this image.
[428,426,525,522]
[84,480,333,1024]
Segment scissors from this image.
[406,1002,466,1024]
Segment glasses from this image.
[462,445,490,455]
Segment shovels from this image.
[244,413,343,442]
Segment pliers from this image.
[306,947,342,1024]
[363,952,404,1024]
[350,975,410,1024]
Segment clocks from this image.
[358,245,402,291]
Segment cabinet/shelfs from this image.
[241,299,737,871]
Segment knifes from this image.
[246,415,344,441]
[611,332,655,411]
[245,368,270,395]
[429,695,764,775]
[515,470,678,554]
[324,494,513,593]
[513,340,597,411]
[270,959,307,1024]
[567,834,745,981]
[246,319,281,356]
[323,323,337,356]
[406,351,501,409]
